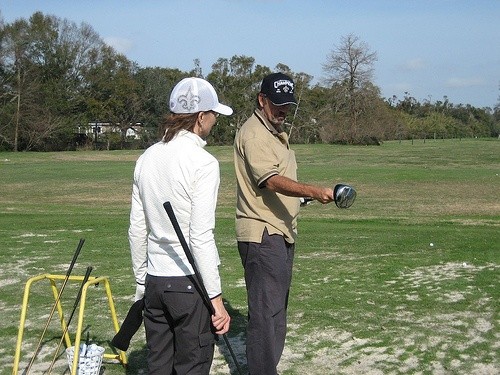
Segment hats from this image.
[260,72,299,106]
[169,77,233,116]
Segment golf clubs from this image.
[25,238,87,374]
[163,200,245,375]
[48,266,92,373]
[302,183,357,210]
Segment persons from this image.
[232,73,335,375]
[127,76,232,375]
